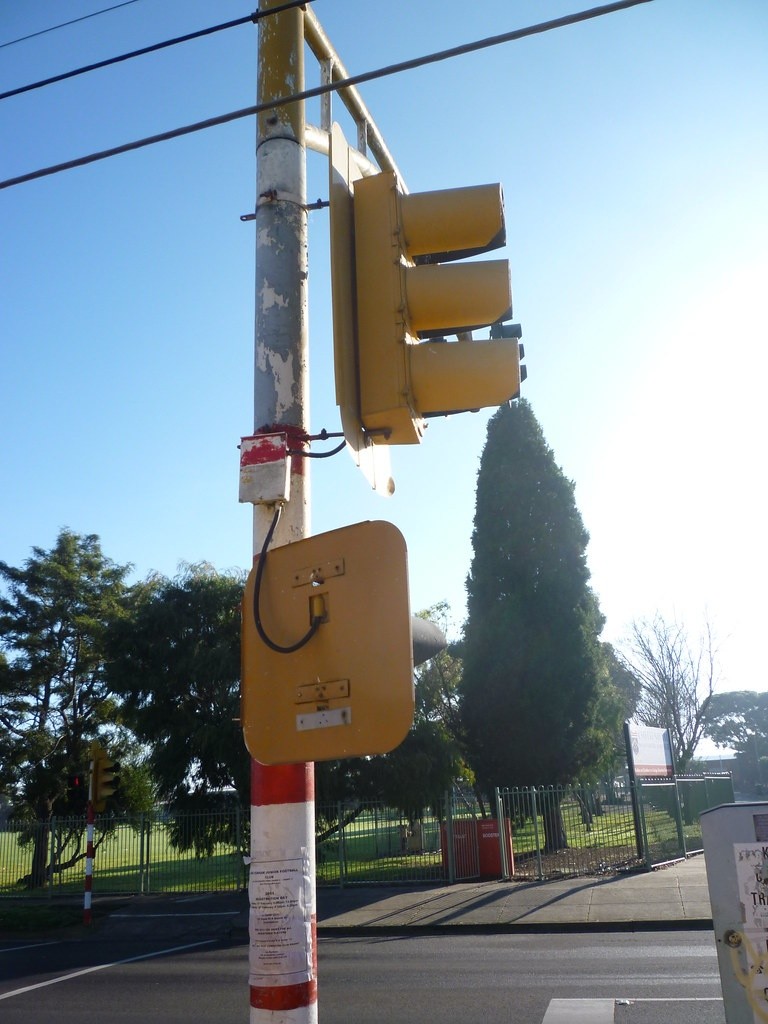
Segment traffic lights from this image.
[92,756,121,812]
[349,169,528,445]
